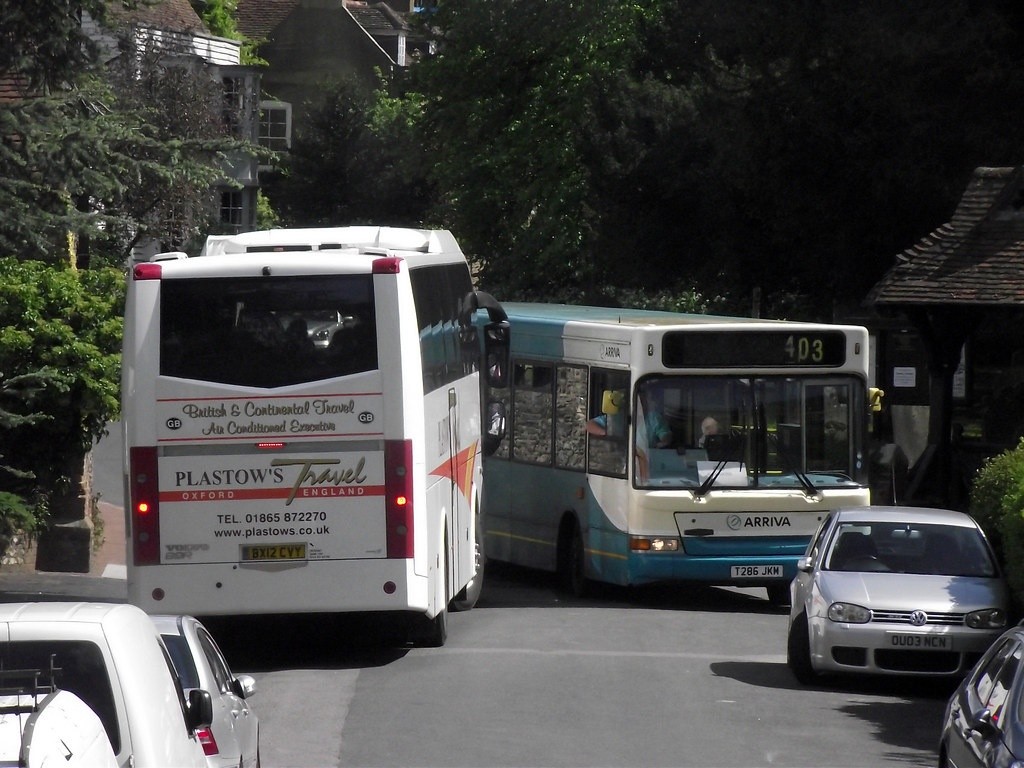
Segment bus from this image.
[476,297,882,609]
[119,225,513,648]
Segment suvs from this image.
[936,616,1024,768]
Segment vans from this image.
[0,600,215,768]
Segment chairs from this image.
[836,532,968,576]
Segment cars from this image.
[1,692,118,768]
[272,307,360,348]
[148,614,261,767]
[784,507,1008,693]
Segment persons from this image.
[583,377,672,470]
[271,319,316,360]
[699,417,717,446]
[488,403,505,436]
